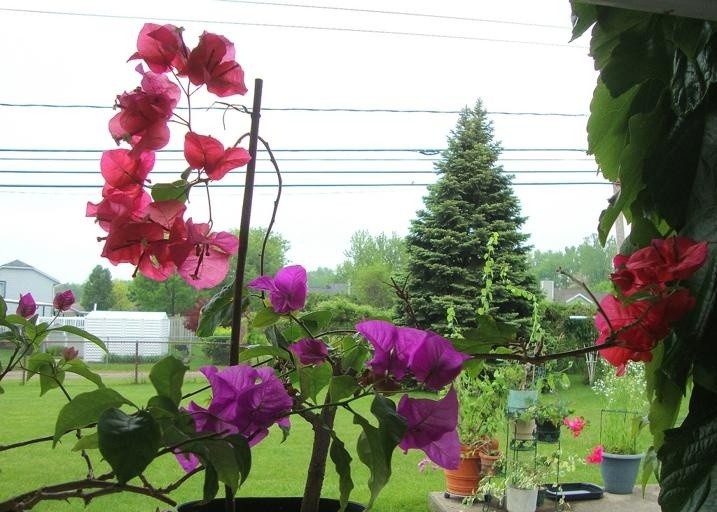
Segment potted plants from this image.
[599,408,644,497]
[418,362,591,512]
